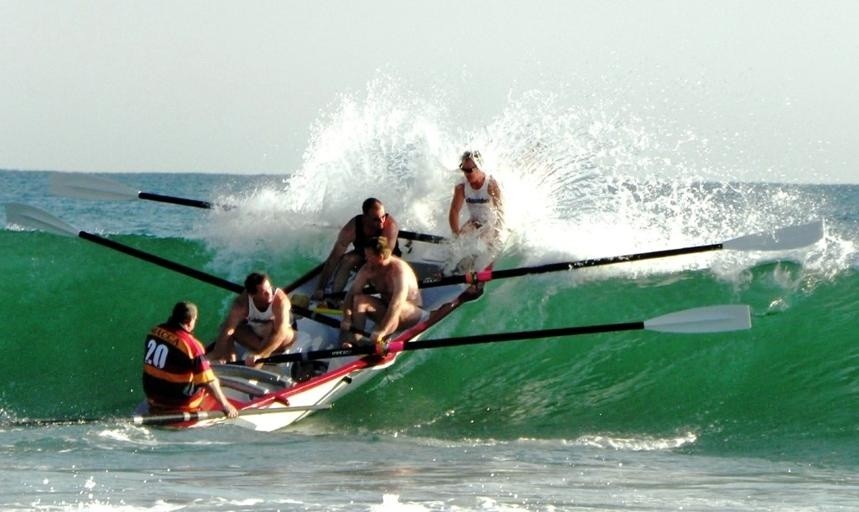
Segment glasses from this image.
[459,162,478,174]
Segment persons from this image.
[212,273,295,367]
[339,236,429,350]
[313,198,402,309]
[142,300,240,419]
[450,150,501,275]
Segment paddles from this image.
[229,305,751,366]
[49,171,452,244]
[326,220,822,297]
[4,200,388,340]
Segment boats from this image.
[126,204,514,452]
